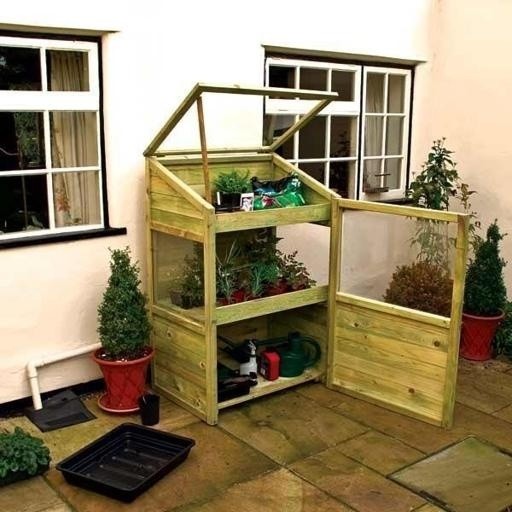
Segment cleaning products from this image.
[239,339,258,374]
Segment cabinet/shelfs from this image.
[144,83,468,428]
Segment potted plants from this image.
[1,426,50,489]
[168,229,317,309]
[459,219,508,361]
[91,247,155,414]
[215,168,254,206]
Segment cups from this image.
[138,394,161,426]
